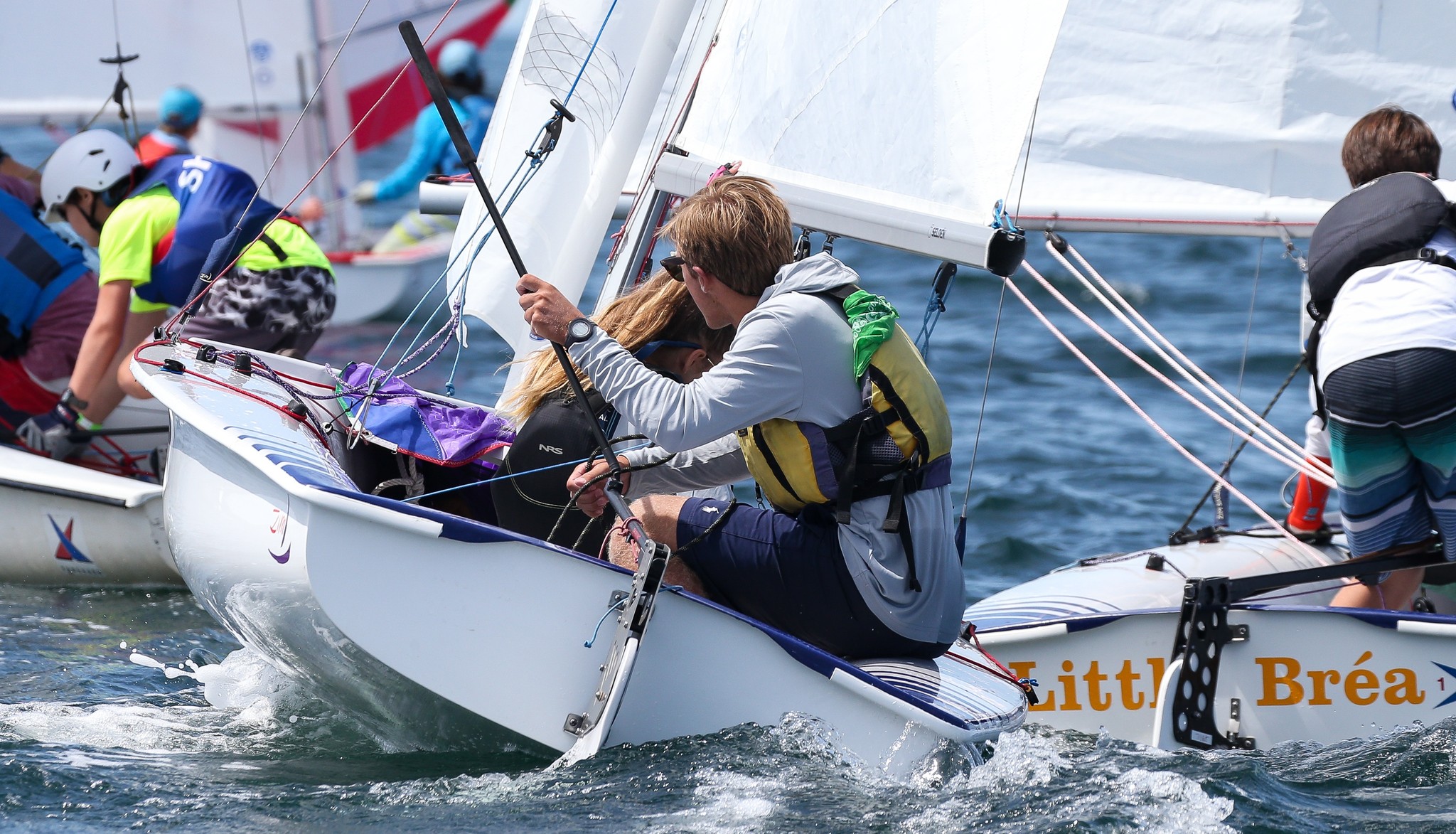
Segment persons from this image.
[351,38,495,209]
[134,85,205,170]
[516,172,967,662]
[491,259,736,564]
[1298,103,1456,616]
[17,127,338,462]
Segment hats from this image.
[159,86,204,132]
[437,38,481,79]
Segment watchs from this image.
[60,389,88,410]
[563,315,598,350]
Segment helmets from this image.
[40,127,140,226]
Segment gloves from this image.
[15,402,92,460]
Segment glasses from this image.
[659,255,685,284]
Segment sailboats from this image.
[0,1,1456,795]
